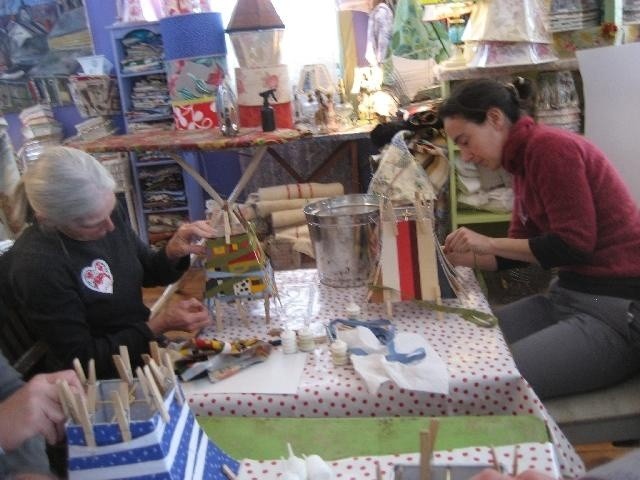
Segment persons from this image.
[439,75,640,399]
[6,145,222,382]
[0,348,86,480]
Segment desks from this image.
[163,264,533,413]
[63,110,382,205]
[188,415,575,480]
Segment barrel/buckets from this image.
[305,194,387,288]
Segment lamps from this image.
[298,64,339,133]
[349,67,385,121]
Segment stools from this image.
[541,376,640,429]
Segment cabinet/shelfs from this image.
[103,12,245,256]
[1,97,135,264]
[437,59,587,296]
